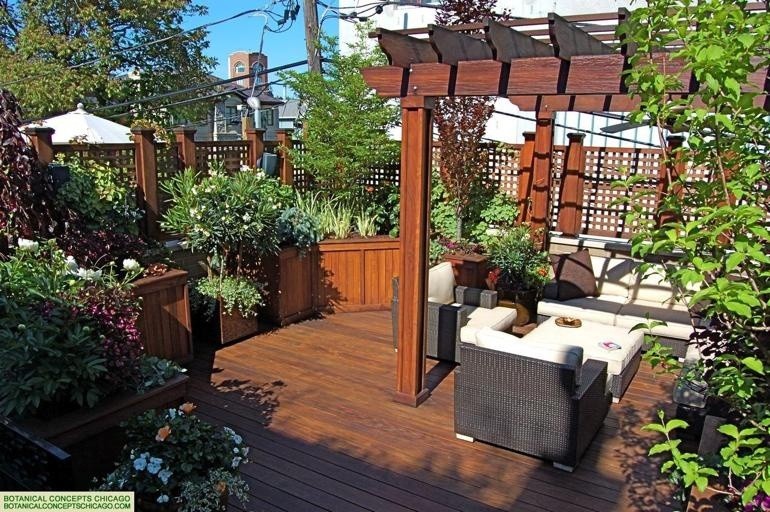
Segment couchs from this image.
[537,251,730,446]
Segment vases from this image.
[216,296,257,344]
[498,287,538,326]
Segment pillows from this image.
[673,267,715,307]
[545,265,556,280]
[628,262,681,304]
[428,261,457,306]
[589,255,634,298]
[548,248,600,302]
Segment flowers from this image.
[481,221,551,292]
[157,158,297,322]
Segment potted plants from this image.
[432,94,495,289]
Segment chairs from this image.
[390,261,518,364]
[453,306,613,473]
